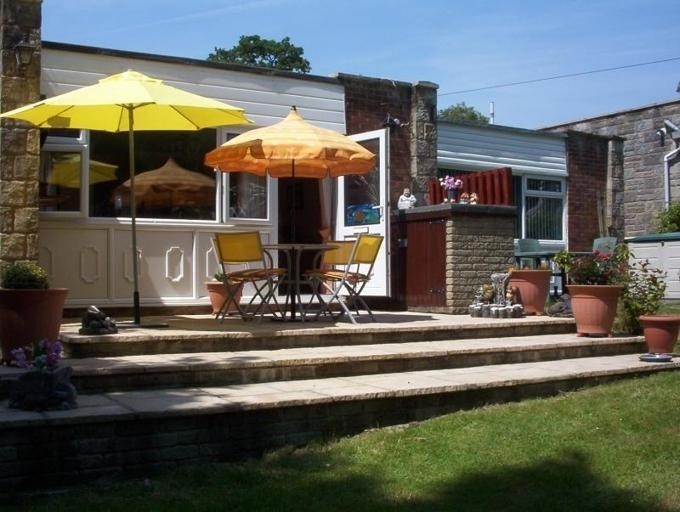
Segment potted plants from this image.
[0,258,69,360]
[621,258,680,353]
[552,248,625,335]
[205,270,244,314]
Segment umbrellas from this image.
[0,68,253,328]
[40,145,117,187]
[110,157,216,208]
[204,115,377,322]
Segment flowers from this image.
[9,339,64,372]
[438,174,463,189]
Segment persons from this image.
[398,188,417,209]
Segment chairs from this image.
[212,229,384,324]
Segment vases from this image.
[9,366,78,410]
[504,268,552,316]
[448,190,457,199]
